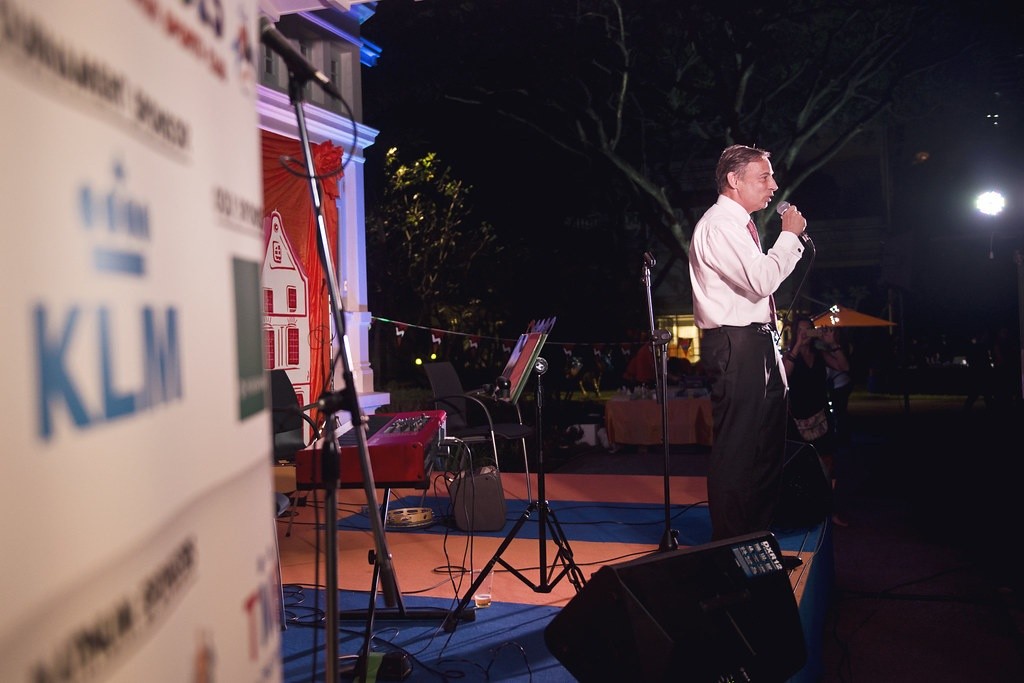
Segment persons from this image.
[825,325,851,412]
[782,314,854,529]
[688,143,808,571]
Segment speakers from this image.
[545,531,808,683]
[451,466,506,532]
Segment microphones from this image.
[777,201,813,249]
[258,17,341,99]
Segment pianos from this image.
[295,410,448,490]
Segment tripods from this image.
[442,331,586,635]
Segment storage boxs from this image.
[573,417,603,447]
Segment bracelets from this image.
[831,346,841,352]
[786,351,799,362]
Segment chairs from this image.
[423,362,532,506]
[270,370,321,538]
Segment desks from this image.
[605,396,714,453]
